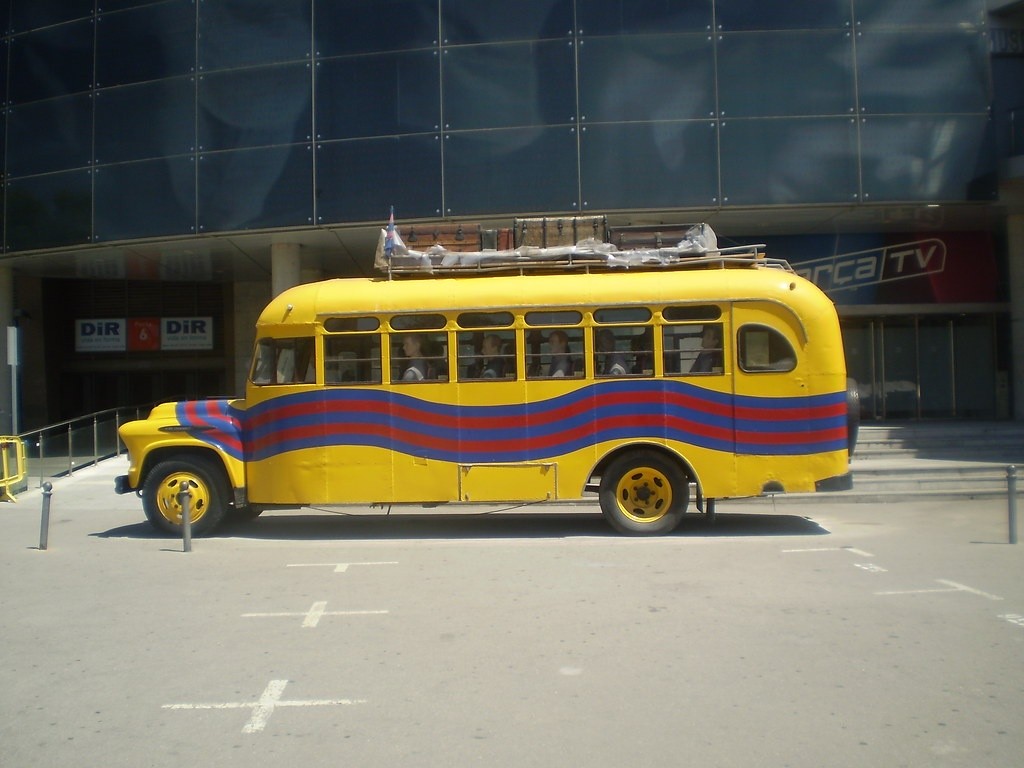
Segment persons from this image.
[477,334,508,379]
[547,331,573,378]
[596,329,631,375]
[687,325,724,374]
[398,332,437,380]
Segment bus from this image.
[112,244,861,538]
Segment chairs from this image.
[527,361,540,375]
[571,357,583,376]
[502,358,516,376]
[427,365,438,379]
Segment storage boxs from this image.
[395,215,696,253]
